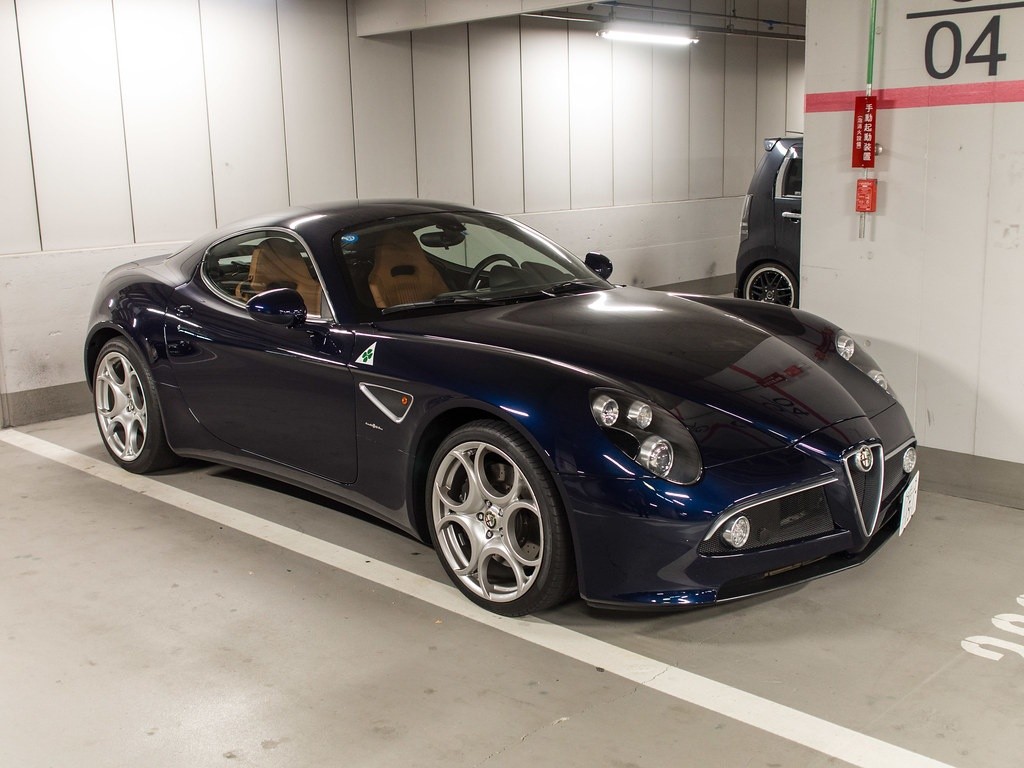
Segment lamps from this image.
[595,20,699,46]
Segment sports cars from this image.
[82,199,922,618]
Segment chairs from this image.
[368,230,449,309]
[236,238,322,316]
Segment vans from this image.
[728,133,801,311]
